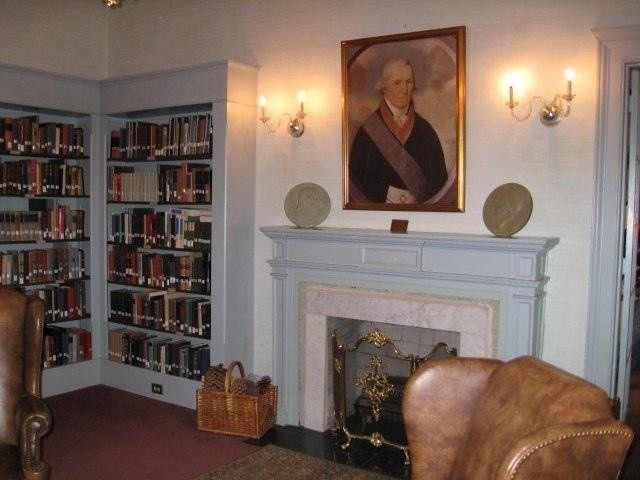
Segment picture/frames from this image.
[339,25,467,214]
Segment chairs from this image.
[1,287,55,480]
[401,354,635,479]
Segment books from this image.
[106,115,212,382]
[1,115,93,368]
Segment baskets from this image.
[196,361,279,441]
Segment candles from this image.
[301,101,303,111]
[567,79,572,96]
[262,106,265,116]
[509,86,514,102]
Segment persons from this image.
[348,56,448,203]
